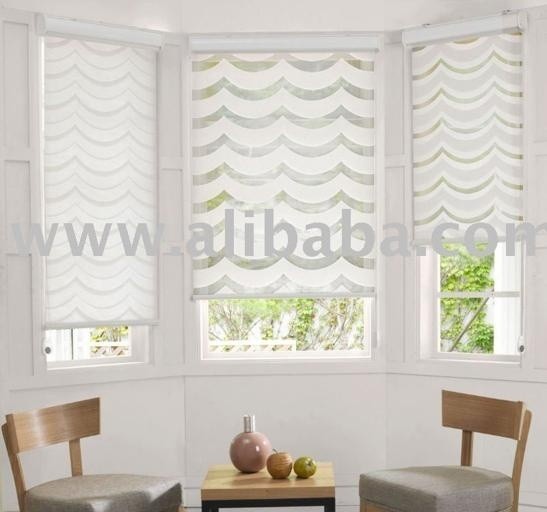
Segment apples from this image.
[267,449,292,478]
[294,456,316,478]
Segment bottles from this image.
[231,414,273,474]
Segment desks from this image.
[200,462,335,511]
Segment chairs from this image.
[359,388,532,511]
[1,397,182,512]
